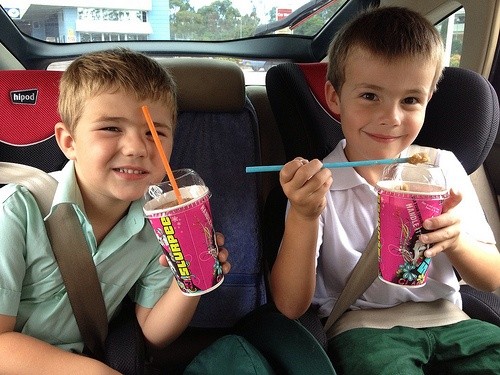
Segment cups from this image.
[373,160,451,289]
[143,167,225,297]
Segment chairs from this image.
[0,65,75,176]
[266,58,499,347]
[131,57,337,375]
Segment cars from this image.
[241,59,275,72]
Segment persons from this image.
[268,4,500,375]
[0,46,231,375]
